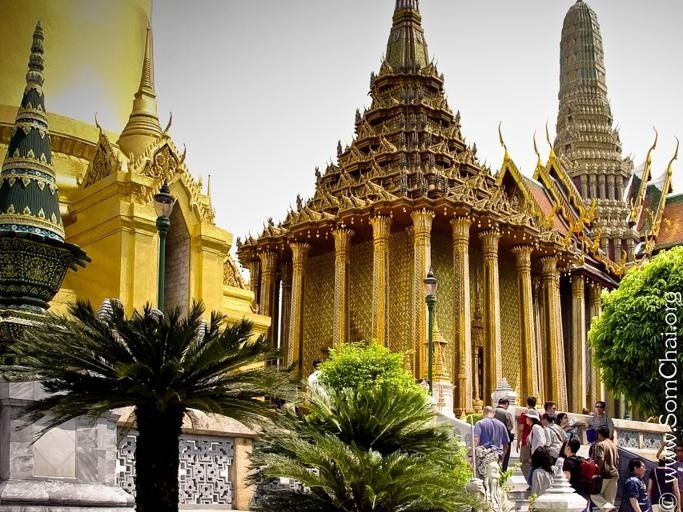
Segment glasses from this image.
[595,406,604,409]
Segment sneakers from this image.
[600,504,617,512]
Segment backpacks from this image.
[567,456,603,495]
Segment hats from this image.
[524,408,541,423]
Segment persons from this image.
[472,397,683,512]
[416,378,438,407]
[308,358,327,395]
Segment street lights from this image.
[151,178,175,317]
[422,265,438,397]
